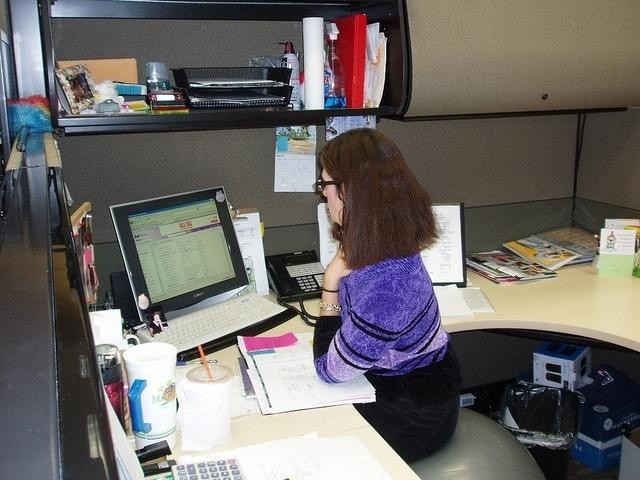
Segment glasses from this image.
[314,177,339,191]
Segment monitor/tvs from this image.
[108,185,250,322]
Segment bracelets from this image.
[318,303,340,312]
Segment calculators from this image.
[171,456,246,480]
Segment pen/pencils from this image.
[197,345,212,378]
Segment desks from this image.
[92,224,639,480]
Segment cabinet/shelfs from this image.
[37,0,409,137]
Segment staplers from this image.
[135,441,177,477]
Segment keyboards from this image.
[137,292,298,361]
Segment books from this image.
[601,213,640,260]
[235,329,377,416]
[330,13,368,107]
[467,220,596,285]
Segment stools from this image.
[406,407,545,479]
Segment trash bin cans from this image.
[497,380,571,480]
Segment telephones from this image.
[265,250,326,326]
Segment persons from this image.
[148,310,165,337]
[313,128,461,465]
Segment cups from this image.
[185,366,233,449]
[122,341,178,440]
